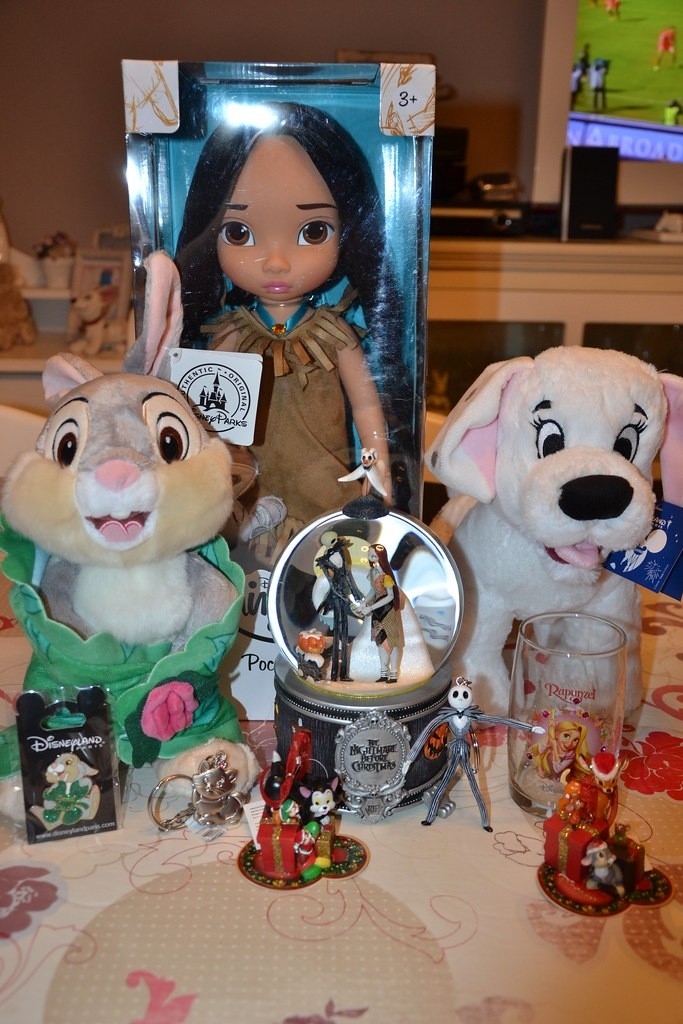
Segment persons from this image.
[570,42,681,128]
[171,99,393,524]
[652,24,677,73]
[401,676,546,833]
[603,0,621,21]
[315,530,405,684]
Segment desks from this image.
[427,240,683,348]
[0,556,683,1024]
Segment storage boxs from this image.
[121,60,424,722]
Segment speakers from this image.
[557,145,620,242]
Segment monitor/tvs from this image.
[531,0,683,244]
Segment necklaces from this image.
[252,302,309,334]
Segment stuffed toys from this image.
[423,346,683,735]
[1,249,260,800]
[69,283,128,355]
[0,260,37,351]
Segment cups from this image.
[507,612,627,818]
